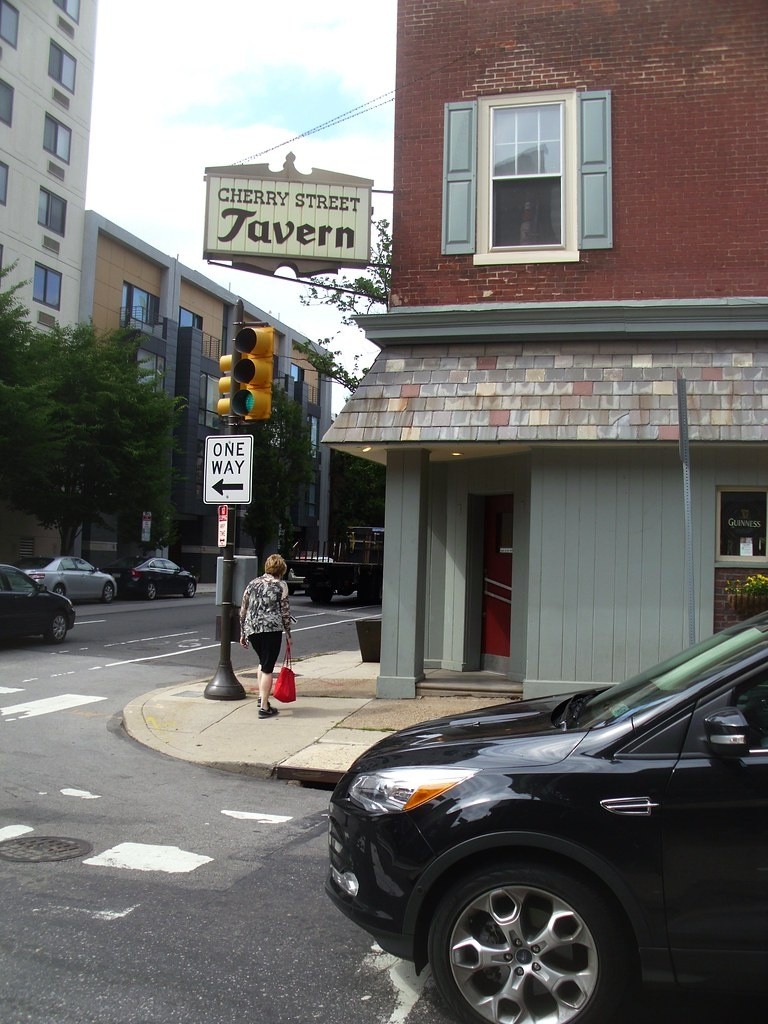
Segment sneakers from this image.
[257,697,270,707]
[258,706,278,718]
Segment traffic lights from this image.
[217,354,232,416]
[232,326,275,421]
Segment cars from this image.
[10,556,197,603]
[0,564,76,643]
[324,610,768,1024]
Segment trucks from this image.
[284,526,385,605]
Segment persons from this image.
[239,554,292,719]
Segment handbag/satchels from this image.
[273,645,296,703]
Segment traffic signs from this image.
[202,434,255,504]
